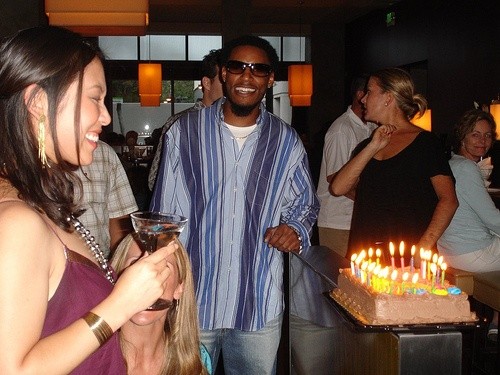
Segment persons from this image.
[148,37,322,374]
[331,67,460,267]
[436,111,500,273]
[316,76,379,258]
[66,141,139,263]
[108,231,208,375]
[486,309,500,335]
[148,49,229,193]
[2,25,180,375]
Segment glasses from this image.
[222,60,272,77]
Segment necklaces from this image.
[58,207,114,285]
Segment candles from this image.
[350,241,447,296]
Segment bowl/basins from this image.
[481,169,493,180]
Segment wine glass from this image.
[130,211,189,311]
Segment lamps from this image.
[409,109,431,132]
[45,0,149,36]
[490,104,500,140]
[138,28,162,107]
[287,0,312,106]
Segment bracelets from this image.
[288,226,303,254]
[81,311,113,346]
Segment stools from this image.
[448,267,500,375]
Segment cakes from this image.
[333,265,477,325]
